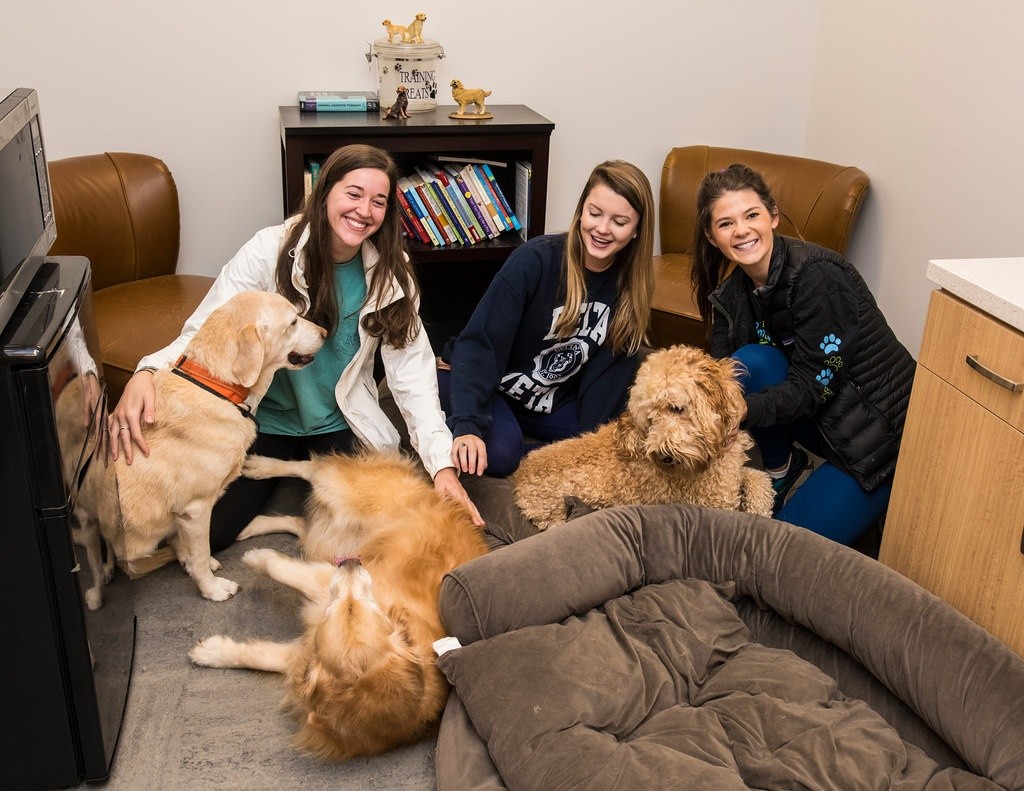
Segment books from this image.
[299,91,380,116]
[302,156,532,246]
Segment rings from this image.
[120,427,130,430]
[461,443,466,446]
[465,498,470,502]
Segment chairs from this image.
[45,151,216,415]
[646,145,870,350]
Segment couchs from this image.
[436,501,1024,791]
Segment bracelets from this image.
[138,370,154,375]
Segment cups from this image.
[366,37,445,114]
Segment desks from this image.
[951,255,952,257]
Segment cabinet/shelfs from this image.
[278,104,556,381]
[877,255,1024,661]
[0,256,138,791]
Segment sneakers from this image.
[771,445,814,516]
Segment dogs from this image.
[509,345,777,532]
[186,449,495,760]
[383,86,413,120]
[381,12,428,45]
[448,80,495,121]
[110,291,330,603]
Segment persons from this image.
[439,160,657,480]
[102,143,486,558]
[687,163,916,547]
